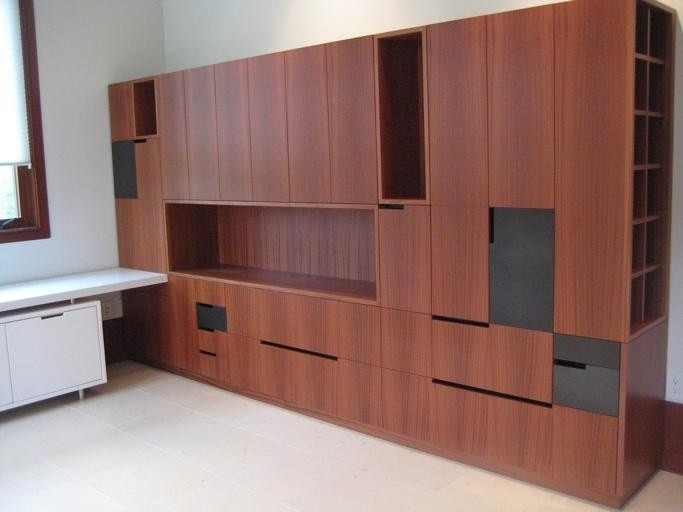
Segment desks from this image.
[0,266,168,413]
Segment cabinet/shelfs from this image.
[107,0,677,508]
